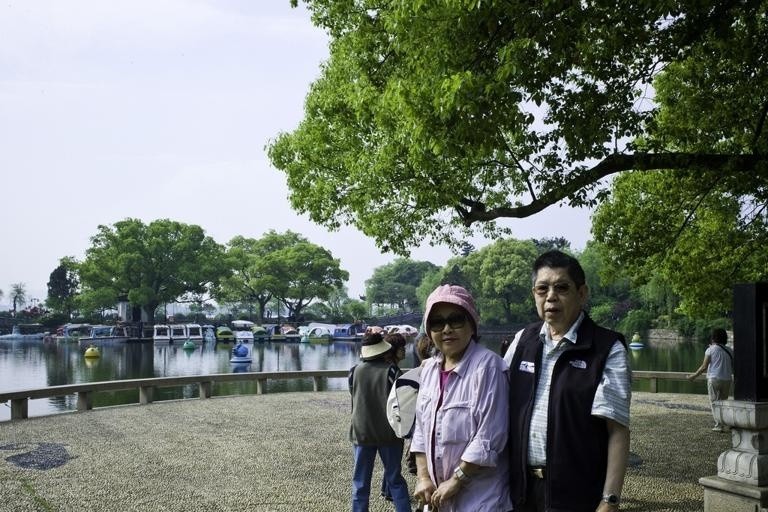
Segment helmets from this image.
[426,309,469,332]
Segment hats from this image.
[359,331,393,359]
[423,284,479,337]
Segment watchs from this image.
[602,493,621,506]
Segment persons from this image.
[504,249,633,512]
[683,327,733,432]
[410,284,514,512]
[345,319,512,512]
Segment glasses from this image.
[532,280,578,294]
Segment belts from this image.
[526,466,547,478]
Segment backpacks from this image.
[386,366,424,439]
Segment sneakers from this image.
[712,426,723,432]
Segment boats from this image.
[629,342,644,349]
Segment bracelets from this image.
[454,467,470,483]
